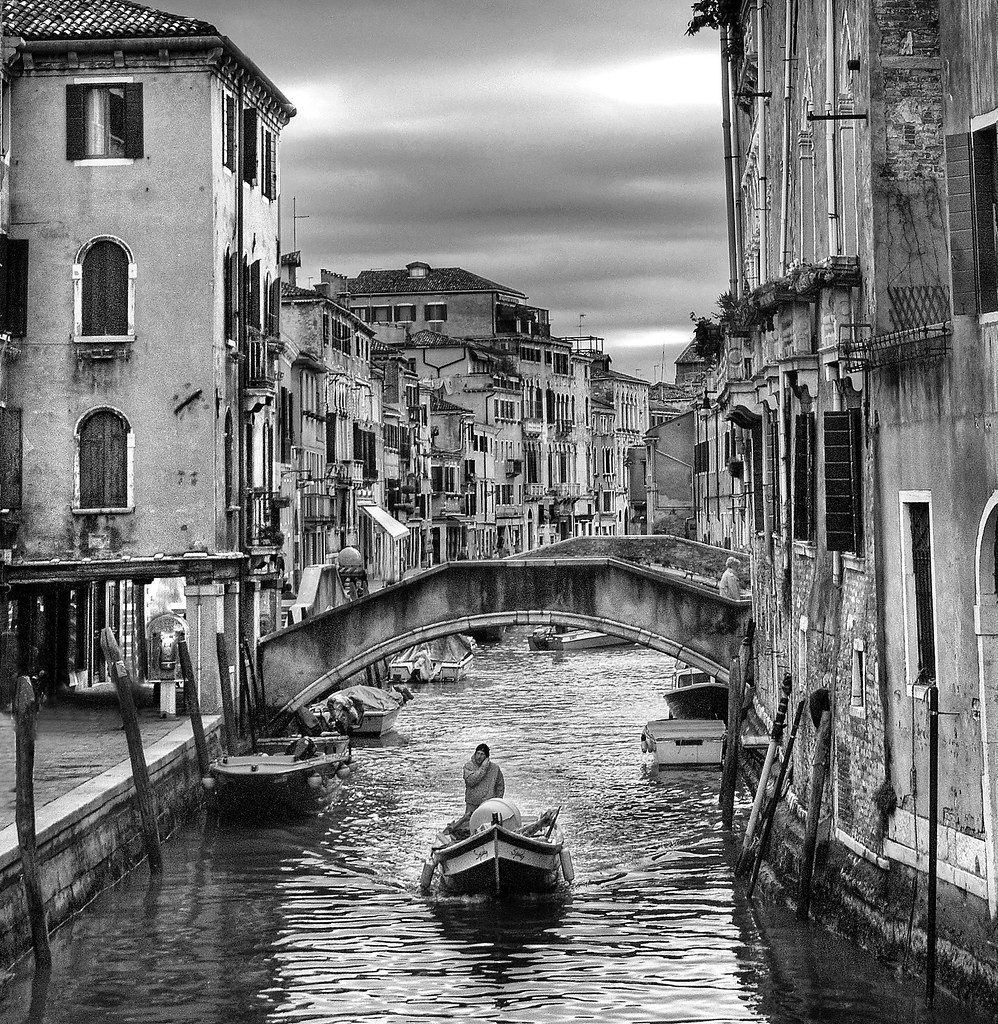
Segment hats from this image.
[476,744,489,757]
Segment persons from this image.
[461,743,505,818]
[718,555,744,603]
[281,583,297,600]
[496,533,505,559]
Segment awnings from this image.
[362,506,410,542]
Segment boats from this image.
[385,632,478,683]
[420,795,560,895]
[526,622,635,650]
[304,684,414,738]
[252,729,353,759]
[640,714,726,767]
[200,751,358,817]
[662,661,728,721]
